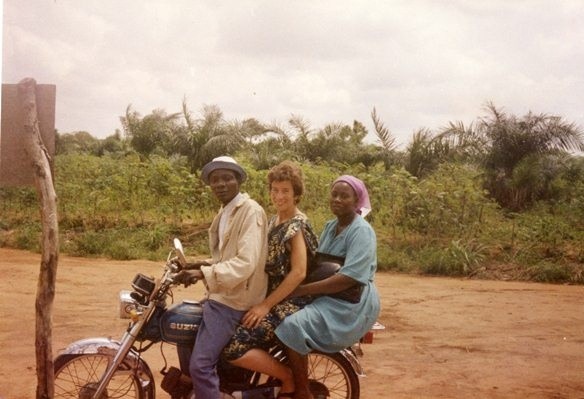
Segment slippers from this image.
[274,388,295,398]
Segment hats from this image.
[201,156,247,184]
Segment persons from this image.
[272,175,381,398]
[170,155,266,398]
[222,160,307,399]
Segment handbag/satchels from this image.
[306,253,367,304]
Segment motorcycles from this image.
[36,237,373,399]
